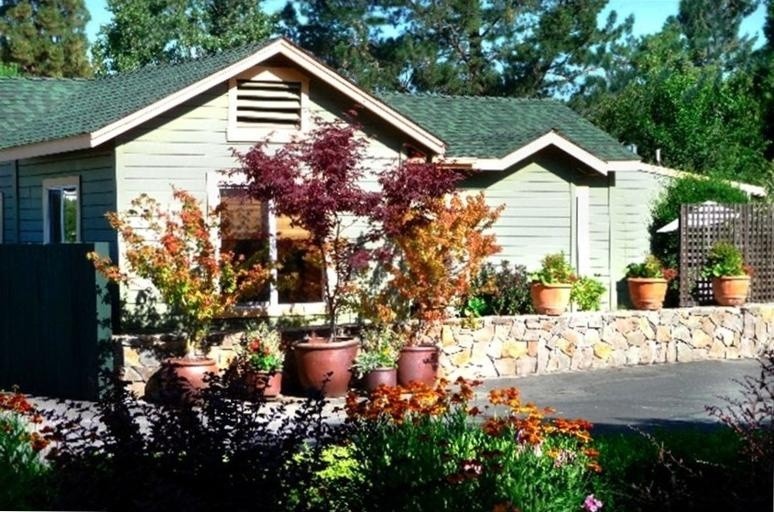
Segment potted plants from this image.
[340,288,409,398]
[233,319,285,400]
[218,118,463,398]
[528,242,753,318]
[385,191,504,391]
[87,183,285,406]
